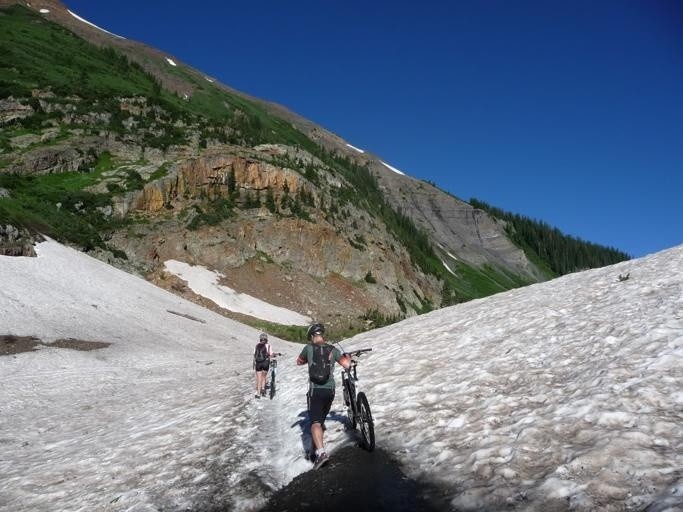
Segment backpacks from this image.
[310,343,334,384]
[255,341,269,362]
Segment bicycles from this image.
[331,348,381,450]
[266,352,287,399]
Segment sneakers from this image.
[310,448,329,471]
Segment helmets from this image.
[260,333,267,341]
[306,324,325,340]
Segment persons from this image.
[297,322,352,473]
[252,333,276,399]
